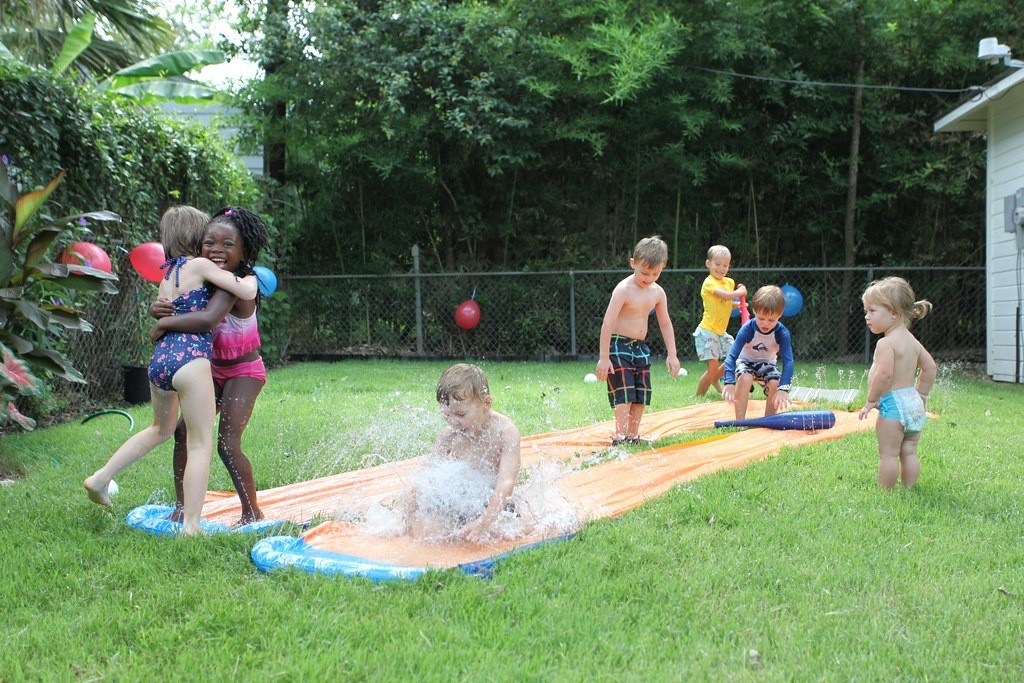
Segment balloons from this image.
[129,242,171,281]
[63,242,111,275]
[781,283,803,318]
[455,301,481,329]
[252,265,277,297]
[731,297,741,318]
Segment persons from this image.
[692,245,750,397]
[405,364,521,545]
[721,285,794,420]
[595,236,682,447]
[859,277,937,488]
[85,206,257,538]
[150,205,266,529]
